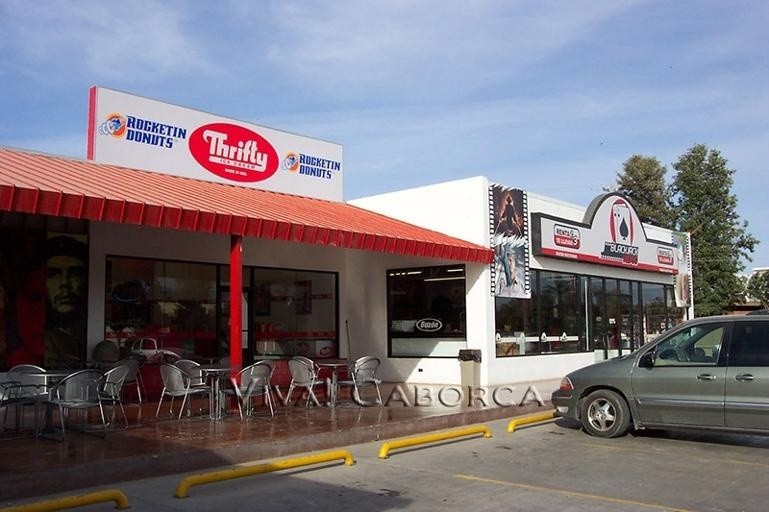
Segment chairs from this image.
[0,341,381,441]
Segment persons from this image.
[1,252,47,372]
[43,234,87,369]
[499,243,519,287]
[497,195,518,236]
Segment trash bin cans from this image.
[459,350,482,387]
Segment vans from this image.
[550,309,769,439]
